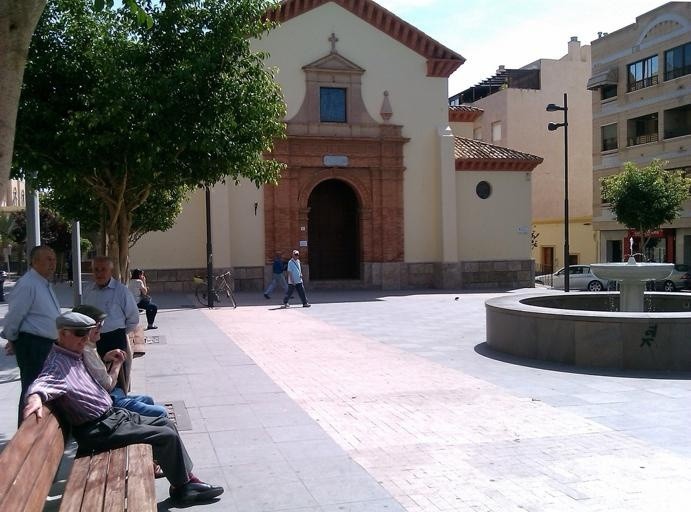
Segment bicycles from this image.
[193,270,238,308]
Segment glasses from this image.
[95,319,105,327]
[69,327,91,338]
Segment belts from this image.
[96,406,116,423]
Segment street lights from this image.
[547,93,571,292]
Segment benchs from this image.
[128,326,146,357]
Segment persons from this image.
[264,249,295,299]
[282,250,311,309]
[22,312,224,508]
[71,305,169,478]
[80,256,139,395]
[0,245,61,429]
[128,268,158,329]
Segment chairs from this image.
[0,404,158,512]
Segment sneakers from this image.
[147,323,158,329]
[263,293,311,308]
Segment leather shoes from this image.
[168,477,226,507]
[152,459,166,478]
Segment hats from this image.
[74,304,108,322]
[293,249,300,256]
[57,311,96,331]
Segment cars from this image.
[536,265,609,292]
[609,264,691,292]
